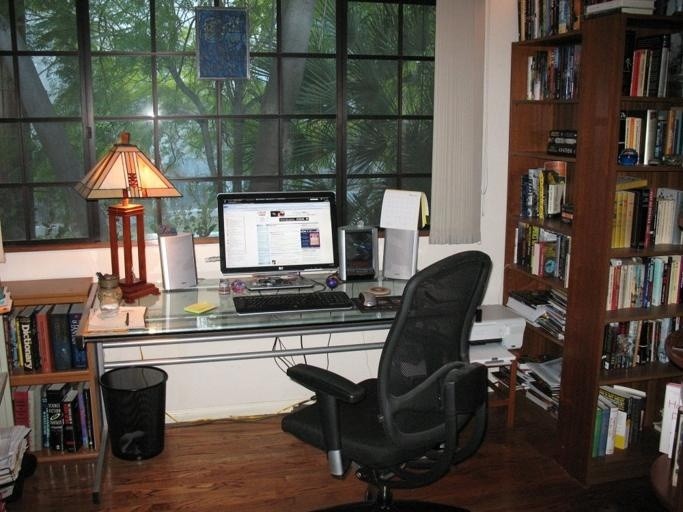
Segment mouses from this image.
[360,290,379,307]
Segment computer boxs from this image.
[337,227,379,281]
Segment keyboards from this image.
[232,291,354,314]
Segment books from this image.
[612,174,683,248]
[489,351,563,413]
[517,0,656,42]
[0,425,30,499]
[87,304,148,333]
[619,106,683,166]
[506,224,572,339]
[0,285,95,453]
[521,129,577,226]
[622,32,683,98]
[526,44,582,99]
[591,382,683,459]
[601,255,683,372]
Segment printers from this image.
[468,304,526,368]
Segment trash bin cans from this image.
[98,366,168,460]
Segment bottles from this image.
[96,272,123,320]
[219,278,230,295]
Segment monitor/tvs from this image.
[217,191,339,291]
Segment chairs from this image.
[282,250,492,512]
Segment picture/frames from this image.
[195,7,250,80]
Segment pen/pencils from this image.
[126,313,129,325]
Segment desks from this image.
[73,272,420,503]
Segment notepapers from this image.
[184,302,218,314]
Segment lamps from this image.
[73,133,184,303]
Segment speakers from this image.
[157,232,196,292]
[385,226,419,281]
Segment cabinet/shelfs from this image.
[501,8,682,489]
[0,277,108,503]
